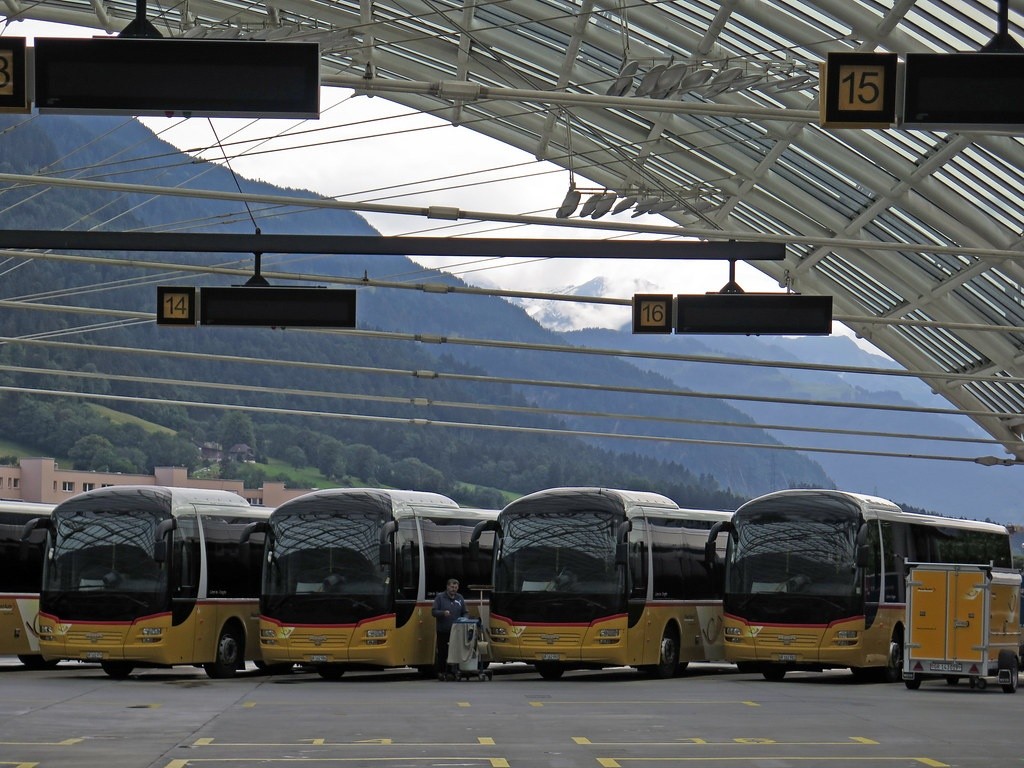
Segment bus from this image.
[23,483,300,677]
[237,484,505,679]
[0,497,86,668]
[466,485,740,680]
[702,486,1015,683]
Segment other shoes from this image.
[438,672,456,681]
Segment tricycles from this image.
[900,560,1021,695]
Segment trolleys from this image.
[446,615,495,683]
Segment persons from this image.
[432,578,470,681]
